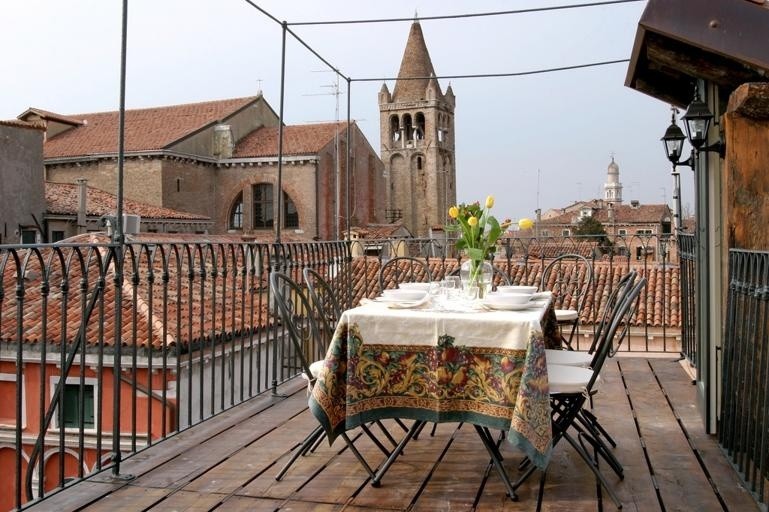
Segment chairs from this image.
[380,257,431,289]
[541,254,592,350]
[545,269,637,471]
[269,272,391,487]
[302,266,412,456]
[431,263,510,437]
[506,277,646,509]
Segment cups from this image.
[439,275,480,311]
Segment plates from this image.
[382,300,423,308]
[487,303,533,310]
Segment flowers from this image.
[445,195,534,291]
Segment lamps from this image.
[679,85,726,158]
[659,113,695,171]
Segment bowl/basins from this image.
[488,286,539,302]
[386,282,437,302]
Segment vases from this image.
[460,260,493,299]
